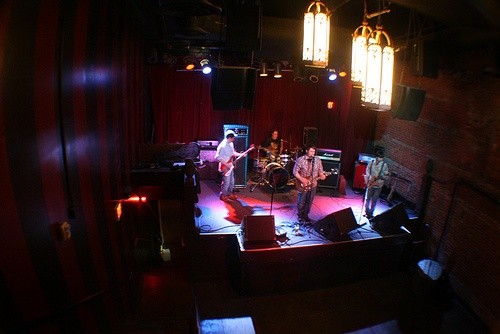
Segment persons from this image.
[213,130,247,200]
[293,143,326,224]
[363,150,388,218]
[261,129,284,156]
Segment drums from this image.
[279,154,291,161]
[253,159,268,168]
[264,162,290,190]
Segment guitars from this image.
[363,172,398,189]
[295,168,340,192]
[218,144,256,177]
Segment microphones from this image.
[312,156,315,165]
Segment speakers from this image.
[310,207,358,242]
[317,162,341,188]
[233,137,248,186]
[396,264,445,334]
[211,61,256,111]
[238,215,276,244]
[369,202,409,236]
[390,83,425,123]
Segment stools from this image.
[378,173,411,207]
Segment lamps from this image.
[185,58,212,75]
[293,0,399,112]
[259,61,282,78]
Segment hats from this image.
[225,130,237,138]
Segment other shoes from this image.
[297,216,312,223]
[220,193,237,200]
